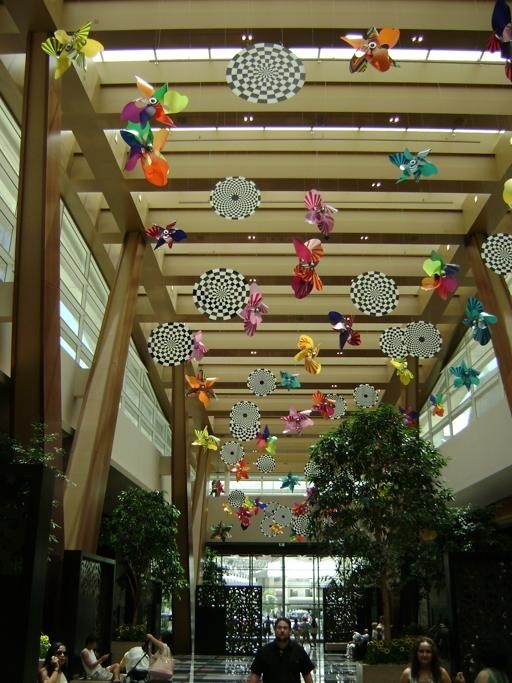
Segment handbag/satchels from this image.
[148,655,175,678]
[126,668,147,681]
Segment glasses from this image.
[55,651,67,654]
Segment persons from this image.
[345,616,384,660]
[227,615,320,682]
[38,631,175,682]
[400,635,511,682]
